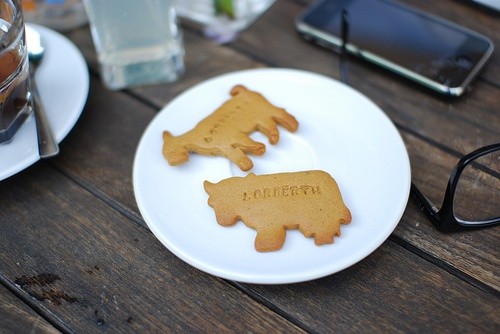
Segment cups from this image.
[0,0,33,146]
[81,2,184,94]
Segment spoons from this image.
[19,21,61,160]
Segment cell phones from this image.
[296,0,493,101]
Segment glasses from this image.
[337,4,500,235]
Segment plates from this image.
[132,66,413,286]
[0,16,93,184]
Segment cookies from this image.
[204,169,353,252]
[162,83,298,172]
